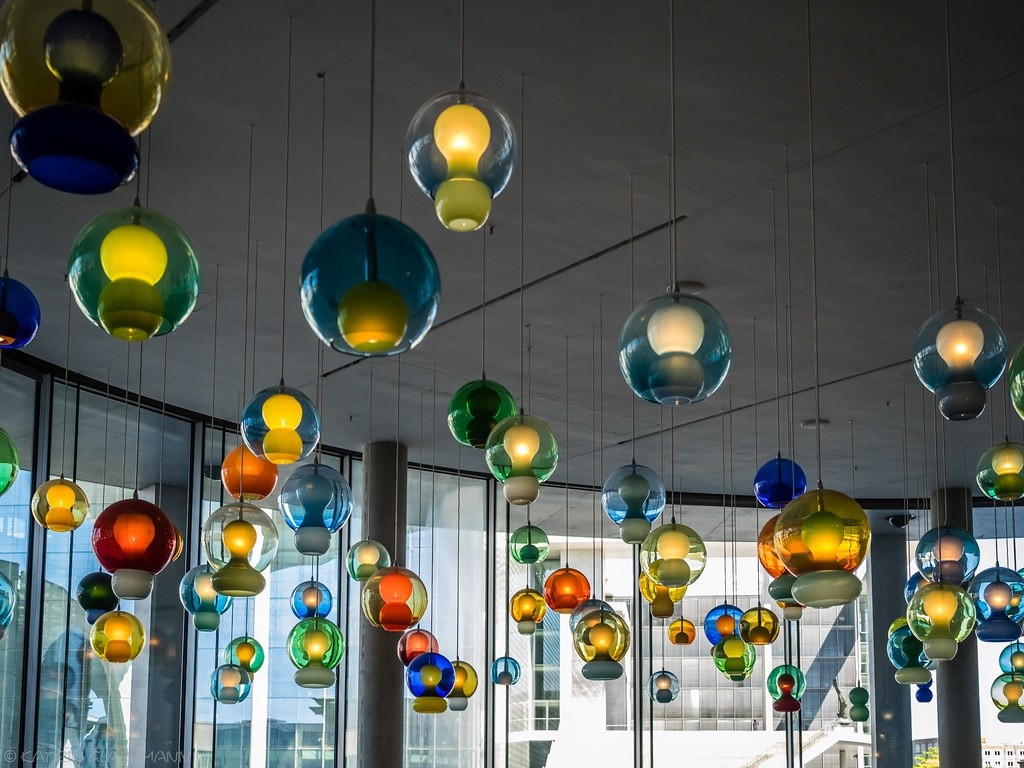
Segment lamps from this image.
[485,73,560,504]
[490,504,520,685]
[398,362,440,674]
[77,242,355,706]
[0,109,42,350]
[509,324,552,565]
[545,0,1024,726]
[0,0,172,195]
[31,283,89,532]
[68,135,200,345]
[446,433,479,710]
[345,361,391,582]
[361,356,429,632]
[403,0,516,232]
[406,362,455,714]
[0,426,19,495]
[300,0,442,360]
[447,222,516,448]
[507,350,548,634]
[0,572,15,641]
